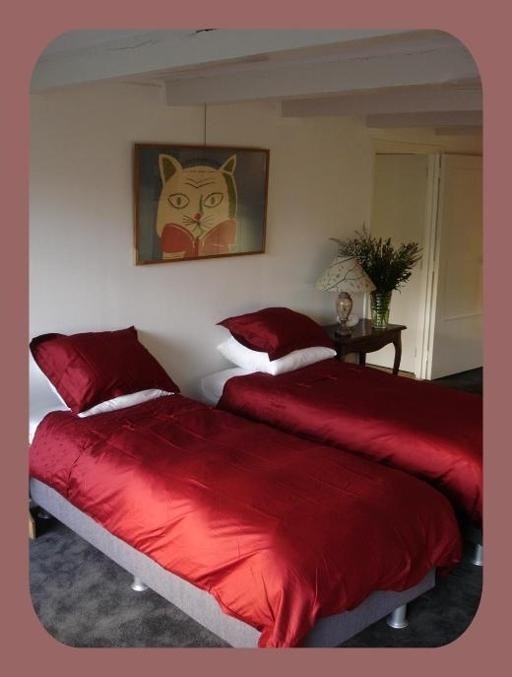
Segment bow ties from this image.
[158,219,237,258]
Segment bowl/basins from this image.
[336,313,360,327]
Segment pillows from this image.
[28,325,181,420]
[211,304,339,377]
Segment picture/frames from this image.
[130,141,271,267]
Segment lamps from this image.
[314,254,375,337]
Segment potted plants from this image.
[328,220,424,329]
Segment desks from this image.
[319,318,408,377]
